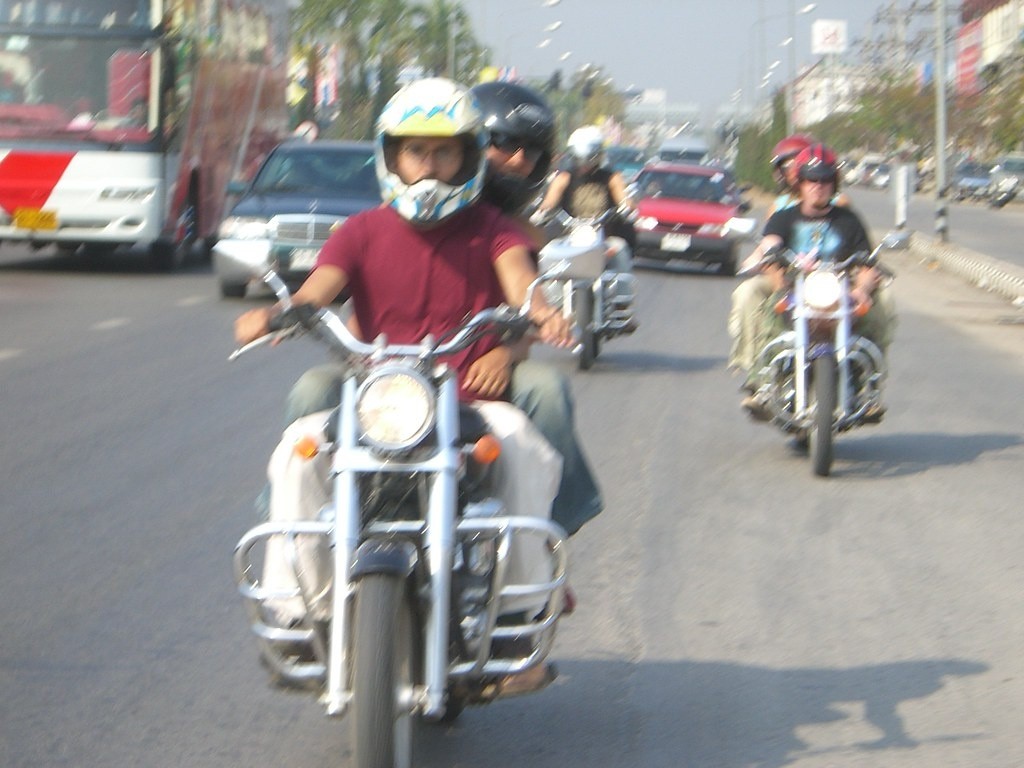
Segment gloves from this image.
[603,213,637,248]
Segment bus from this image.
[1,0,284,275]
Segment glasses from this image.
[492,133,543,164]
[807,177,833,184]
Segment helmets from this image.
[566,126,608,177]
[770,135,815,172]
[375,77,489,227]
[469,83,556,213]
[794,144,838,179]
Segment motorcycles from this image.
[210,223,610,767]
[717,215,913,477]
[527,183,644,370]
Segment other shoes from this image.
[562,591,576,615]
[741,397,772,422]
[275,620,328,691]
[863,401,887,419]
[495,612,547,691]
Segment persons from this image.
[274,83,606,615]
[236,75,580,688]
[742,145,887,423]
[538,126,636,250]
[768,133,853,219]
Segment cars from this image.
[599,135,711,191]
[835,149,1024,206]
[627,163,754,276]
[210,140,381,300]
